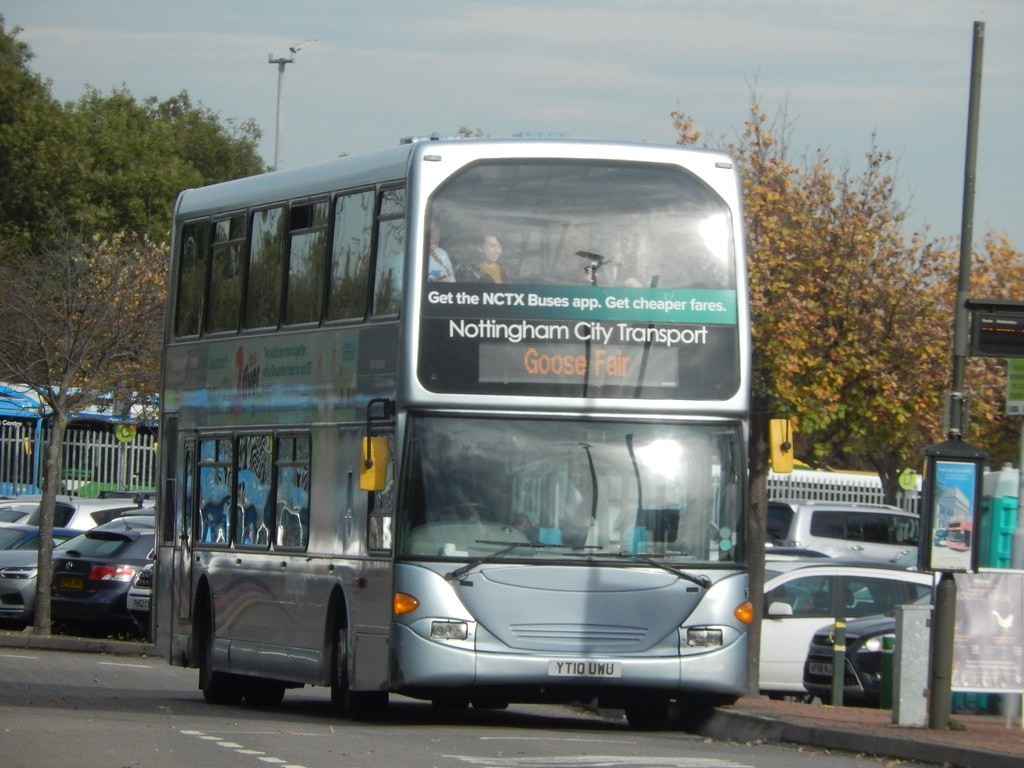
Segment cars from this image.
[750,498,1011,700]
[0,487,158,638]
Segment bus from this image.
[149,134,798,734]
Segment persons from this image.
[576,241,643,289]
[428,224,456,283]
[459,230,513,284]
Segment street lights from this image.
[268,46,301,171]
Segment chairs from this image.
[813,590,855,615]
